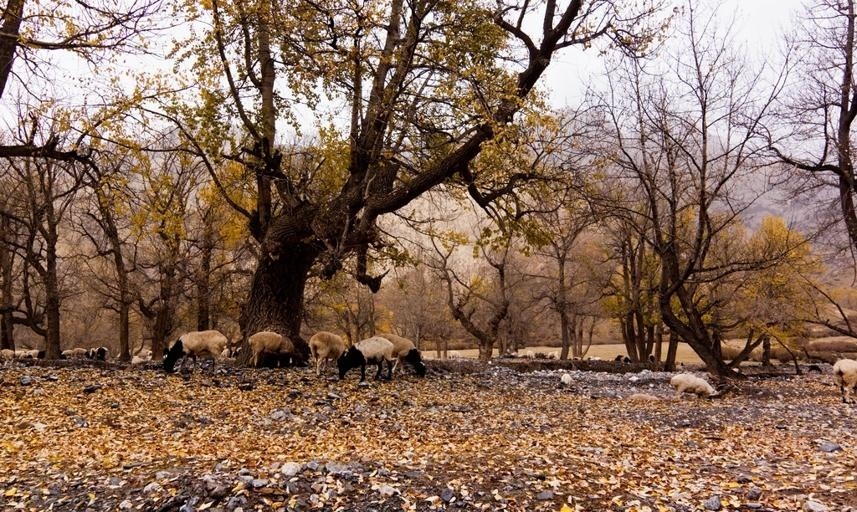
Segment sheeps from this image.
[61,345,110,363]
[832,359,857,404]
[375,332,428,379]
[309,330,350,377]
[337,334,400,384]
[247,330,303,369]
[670,372,719,400]
[117,346,153,366]
[160,329,228,374]
[0,348,44,367]
